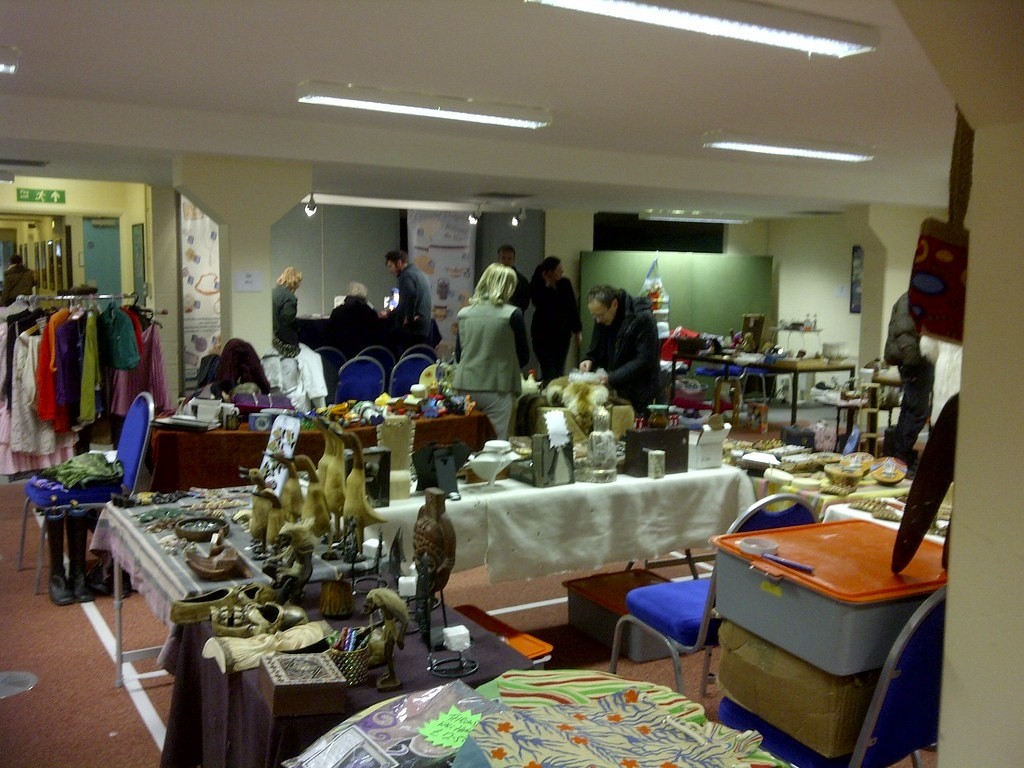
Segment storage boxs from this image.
[782,424,814,449]
[748,403,769,436]
[563,569,683,663]
[625,422,732,476]
[259,652,346,718]
[454,603,553,670]
[708,518,948,761]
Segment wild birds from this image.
[234,413,389,551]
[412,487,458,655]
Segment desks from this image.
[151,409,490,492]
[821,503,950,545]
[672,351,901,459]
[750,474,913,522]
[93,463,757,768]
[297,318,443,352]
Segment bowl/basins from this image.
[734,536,779,557]
[813,451,908,488]
[722,439,844,474]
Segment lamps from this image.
[296,0,879,218]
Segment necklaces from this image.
[273,333,299,357]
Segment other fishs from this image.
[387,527,406,582]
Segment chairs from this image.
[17,390,155,594]
[607,494,824,700]
[698,361,747,409]
[313,344,439,402]
[716,584,948,768]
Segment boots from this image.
[66,509,95,602]
[46,508,74,606]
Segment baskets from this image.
[331,640,371,686]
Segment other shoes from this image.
[87,563,131,596]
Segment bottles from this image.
[803,313,817,330]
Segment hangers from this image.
[1,291,163,336]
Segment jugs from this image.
[647,405,670,429]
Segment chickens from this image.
[520,369,538,394]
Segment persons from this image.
[530,255,583,389]
[389,287,399,311]
[450,262,530,442]
[497,244,530,314]
[579,283,659,419]
[1,254,36,306]
[379,250,433,344]
[272,267,302,342]
[884,292,963,464]
[327,281,378,353]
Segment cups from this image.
[248,407,293,432]
[184,399,240,430]
[321,580,354,617]
[330,643,369,685]
[648,449,665,480]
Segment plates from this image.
[175,517,229,541]
